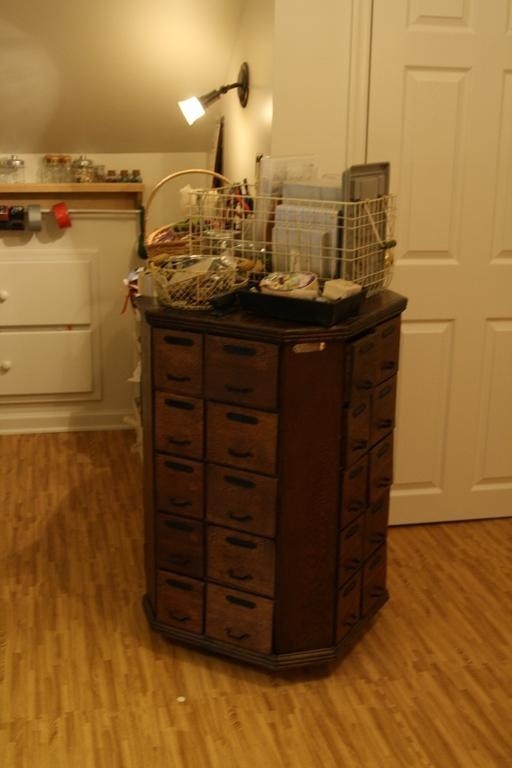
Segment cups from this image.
[1,203,73,232]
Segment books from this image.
[270,203,341,281]
[166,257,217,288]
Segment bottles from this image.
[4,153,142,184]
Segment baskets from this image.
[142,166,254,313]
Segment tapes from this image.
[0,203,72,232]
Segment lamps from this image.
[177,61,250,127]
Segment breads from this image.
[261,270,318,299]
[324,279,361,299]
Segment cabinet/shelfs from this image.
[0,251,103,406]
[132,291,411,675]
